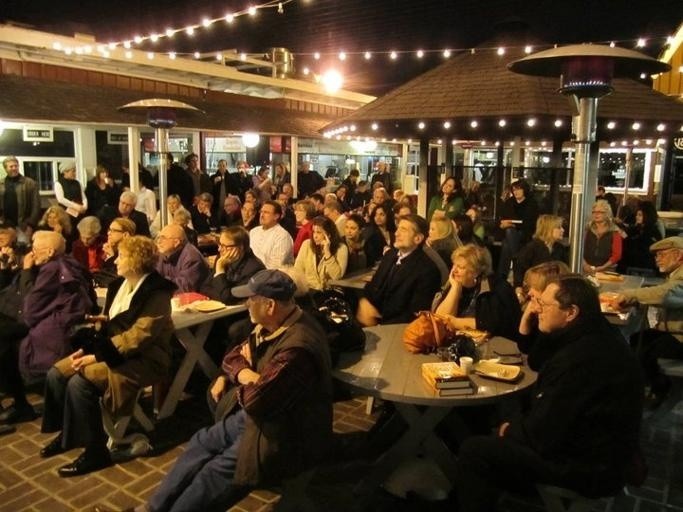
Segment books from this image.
[424,362,475,397]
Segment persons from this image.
[40,236,176,473]
[309,193,325,213]
[427,215,464,269]
[93,218,136,288]
[364,205,397,268]
[105,192,151,238]
[0,156,40,239]
[452,215,485,249]
[235,202,260,231]
[624,211,663,272]
[186,153,208,203]
[282,184,293,197]
[584,200,622,274]
[37,206,72,245]
[253,165,274,201]
[94,271,333,512]
[366,162,390,211]
[292,203,321,253]
[55,159,88,227]
[1,230,94,436]
[222,197,243,225]
[432,243,521,338]
[427,177,464,220]
[244,190,262,210]
[324,169,368,207]
[323,203,351,238]
[152,225,211,292]
[192,193,219,233]
[393,190,416,223]
[340,214,376,273]
[295,215,349,302]
[173,209,197,245]
[156,153,192,203]
[276,192,297,238]
[466,208,486,240]
[358,214,442,326]
[121,160,154,192]
[458,273,645,512]
[85,164,119,222]
[138,172,157,227]
[0,228,36,396]
[499,182,539,265]
[609,237,683,410]
[250,201,294,271]
[513,214,567,302]
[151,194,194,234]
[69,217,107,272]
[200,226,267,362]
[231,160,253,203]
[520,262,571,370]
[208,160,231,207]
[598,187,617,208]
[275,162,291,193]
[299,162,324,196]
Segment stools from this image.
[99,386,154,452]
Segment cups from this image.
[460,356,473,377]
[171,298,179,309]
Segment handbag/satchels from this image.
[404,311,455,353]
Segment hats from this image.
[479,336,522,365]
[58,159,75,175]
[649,236,683,251]
[232,269,297,300]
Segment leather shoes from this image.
[41,432,64,457]
[59,451,111,477]
[0,424,15,434]
[1,404,35,423]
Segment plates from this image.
[478,361,521,381]
[195,299,226,311]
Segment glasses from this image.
[592,211,606,214]
[536,298,572,308]
[33,246,49,253]
[217,242,237,250]
[107,228,124,232]
[655,249,677,258]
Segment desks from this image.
[587,271,645,325]
[95,287,249,419]
[328,323,538,481]
[328,261,381,289]
